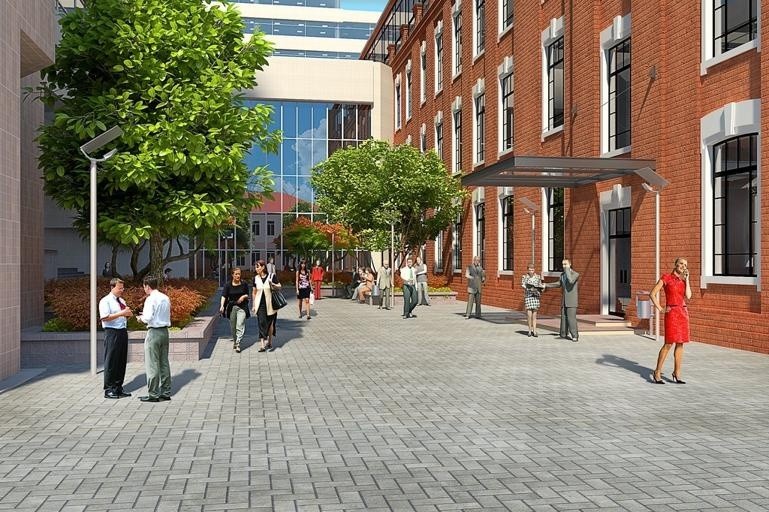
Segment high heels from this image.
[652,371,665,384]
[671,372,686,384]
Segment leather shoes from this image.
[234,344,272,352]
[528,332,578,341]
[104,389,171,402]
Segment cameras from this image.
[126,307,142,319]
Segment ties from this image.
[116,297,125,310]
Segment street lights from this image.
[517,196,540,265]
[79,125,124,376]
[633,165,671,341]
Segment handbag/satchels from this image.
[272,289,288,310]
